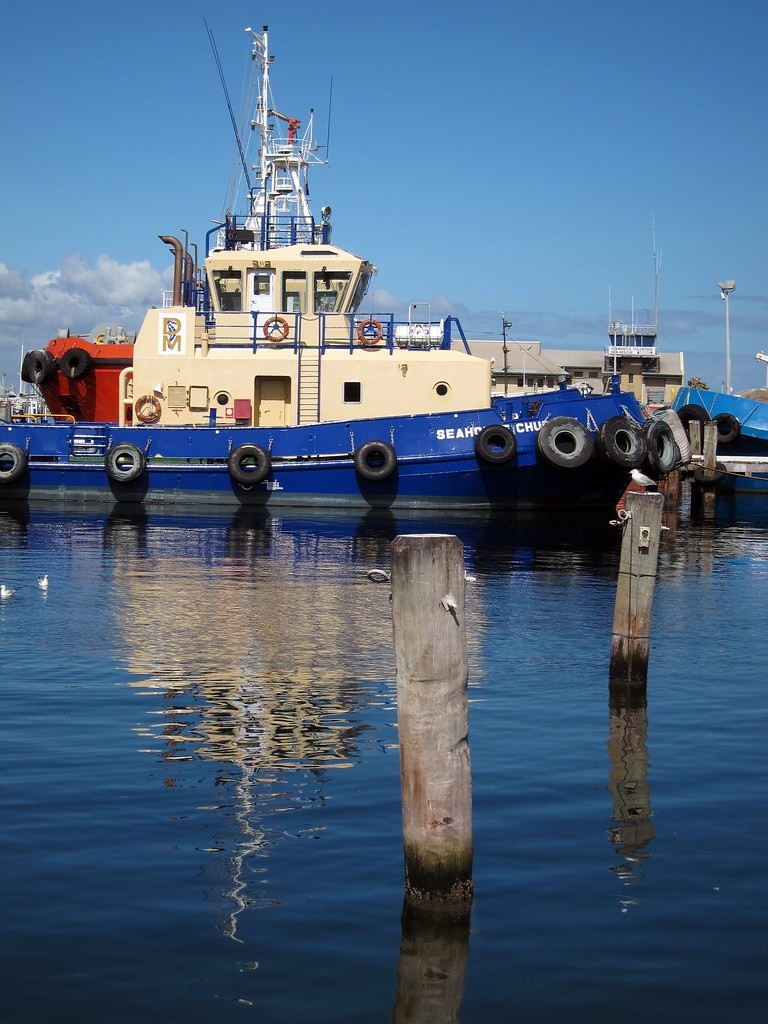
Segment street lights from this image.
[719,279,735,395]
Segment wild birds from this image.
[628,469,658,493]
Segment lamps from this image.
[321,205,331,221]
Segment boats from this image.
[668,385,768,495]
[0,18,679,512]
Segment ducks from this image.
[38,575,50,586]
[0,585,17,598]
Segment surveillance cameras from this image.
[721,292,726,300]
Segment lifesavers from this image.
[135,395,162,422]
[537,416,594,469]
[0,441,27,482]
[104,442,146,481]
[712,413,741,443]
[61,348,93,381]
[354,441,397,481]
[694,463,727,484]
[677,404,711,443]
[597,416,647,469]
[476,424,518,464]
[358,319,383,345]
[21,350,35,384]
[643,420,677,474]
[262,317,290,341]
[229,443,273,484]
[27,350,56,385]
